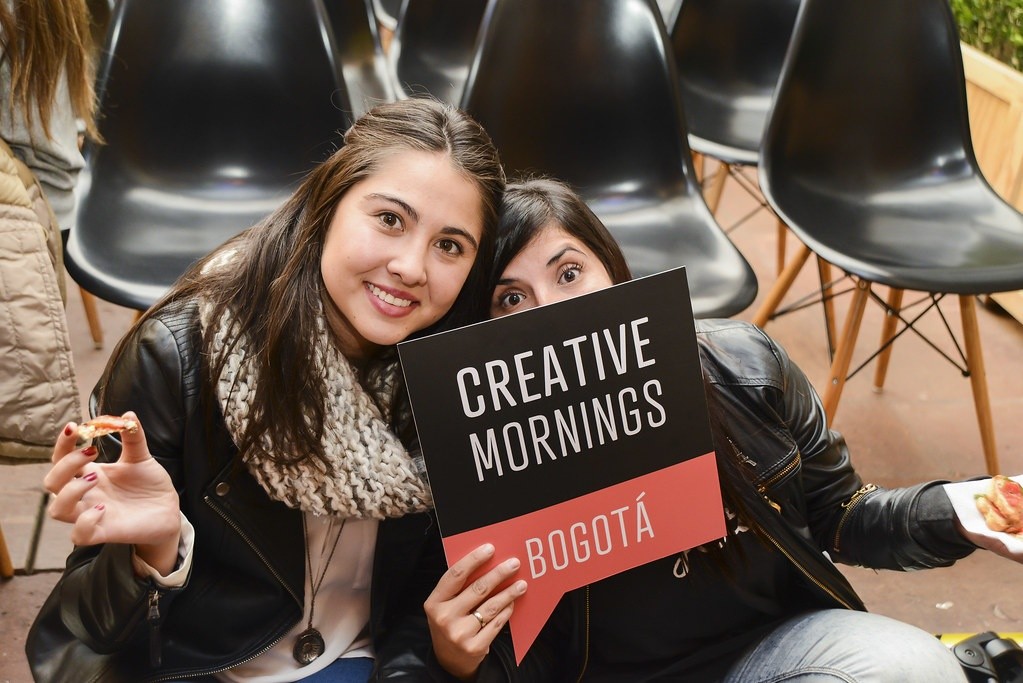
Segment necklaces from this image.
[292,511,347,666]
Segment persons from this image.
[421,171,1023,683]
[24,82,507,683]
[0,0,110,258]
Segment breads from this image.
[975,475,1023,534]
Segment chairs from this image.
[456,0,759,319]
[325,0,492,118]
[66,0,355,312]
[668,0,836,358]
[756,0,1023,475]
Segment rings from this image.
[472,611,486,628]
